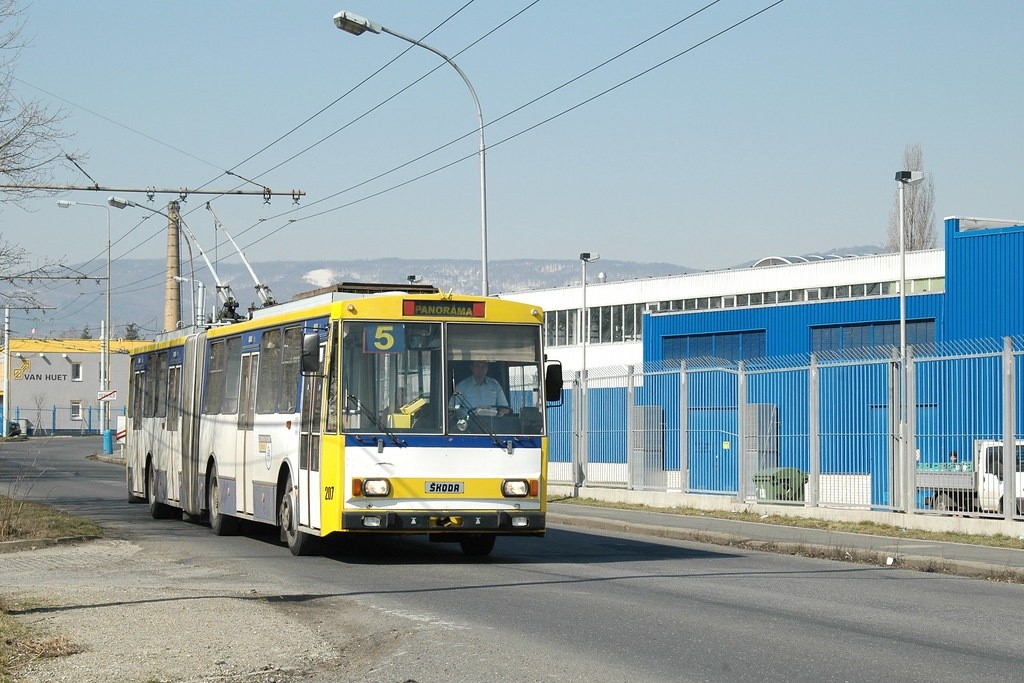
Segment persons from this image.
[449,361,509,417]
[950,451,957,463]
[381,388,408,427]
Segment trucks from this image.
[913,437,1024,519]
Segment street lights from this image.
[577,252,602,485]
[894,170,927,510]
[171,272,205,332]
[333,9,489,303]
[56,199,110,429]
[108,196,197,326]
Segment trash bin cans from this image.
[752,467,809,502]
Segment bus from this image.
[125,200,565,562]
